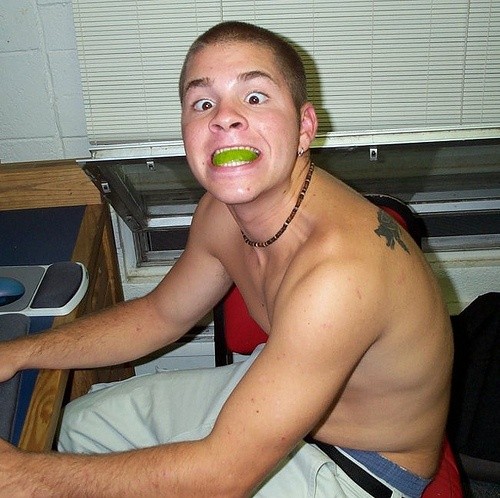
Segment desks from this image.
[0,158,135,498]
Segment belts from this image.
[303,434,393,498]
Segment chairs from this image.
[213,195,463,498]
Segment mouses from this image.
[0,276,25,307]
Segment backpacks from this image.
[446,292,500,456]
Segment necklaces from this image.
[238,161,315,247]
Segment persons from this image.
[0,21,453,498]
